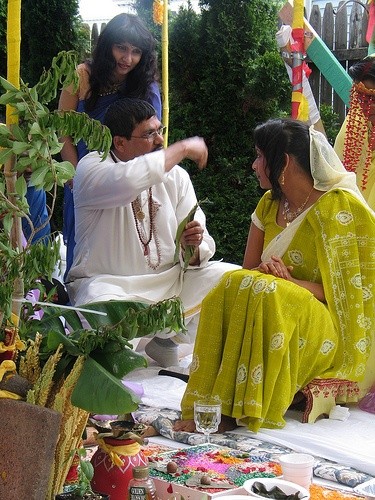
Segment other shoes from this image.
[146,337,180,367]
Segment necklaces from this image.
[282,189,314,227]
[109,152,161,269]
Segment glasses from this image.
[121,125,166,143]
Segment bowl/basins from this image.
[243,478,310,500]
[94,420,148,438]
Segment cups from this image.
[279,454,314,489]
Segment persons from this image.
[19,151,70,307]
[53,13,162,280]
[170,54,375,435]
[63,98,242,368]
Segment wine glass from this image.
[194,400,221,443]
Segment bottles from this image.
[128,466,159,500]
